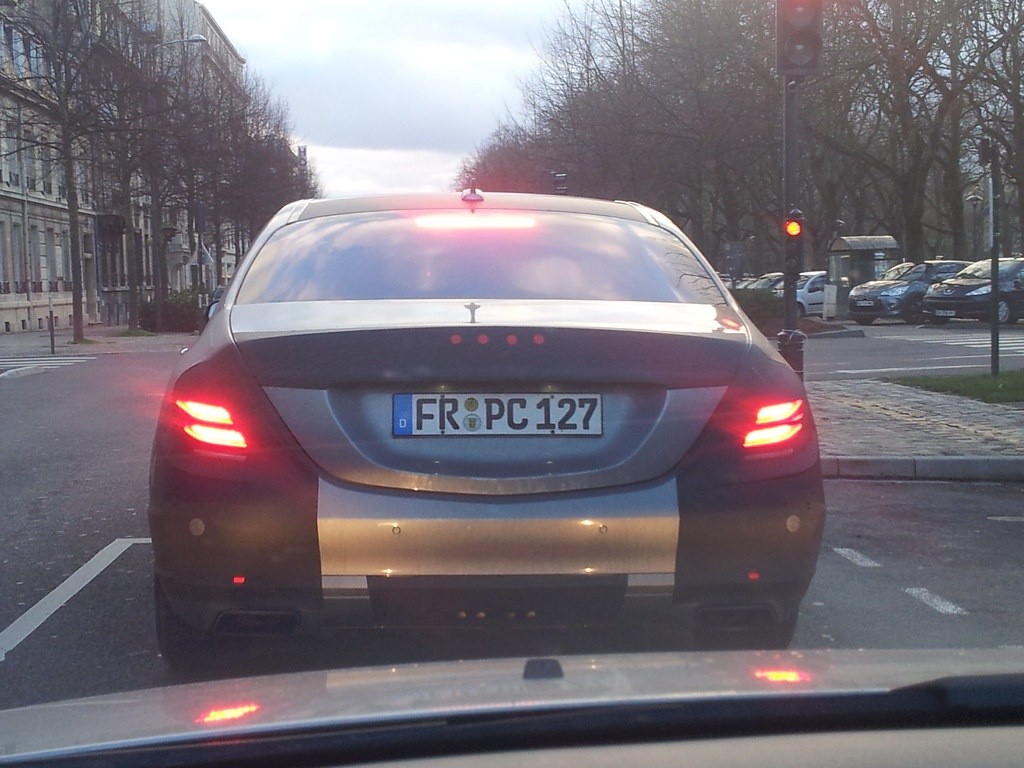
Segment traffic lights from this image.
[776,0,826,78]
[783,218,804,272]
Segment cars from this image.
[921,257,1024,325]
[717,272,784,290]
[875,259,917,281]
[770,269,849,320]
[144,178,828,669]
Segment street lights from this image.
[965,195,984,262]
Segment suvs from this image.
[848,260,975,326]
